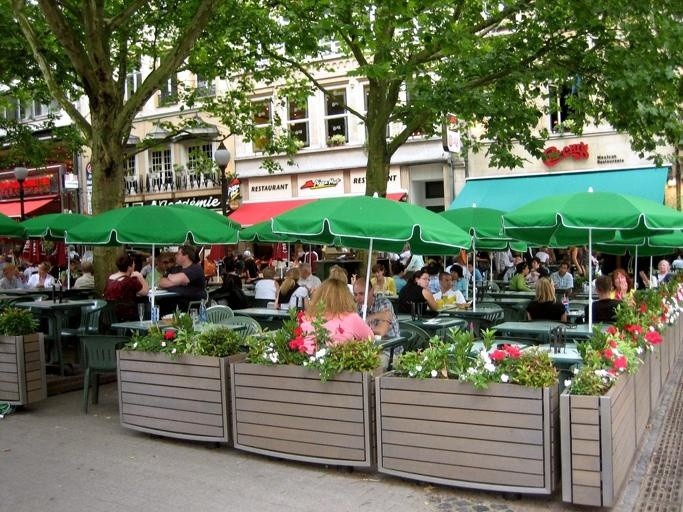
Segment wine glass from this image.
[136,302,146,327]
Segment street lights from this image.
[15,162,28,223]
[212,138,231,215]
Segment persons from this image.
[91,252,149,323]
[351,279,404,359]
[584,275,628,324]
[634,261,678,288]
[296,276,375,359]
[371,251,491,318]
[609,268,636,308]
[526,278,567,321]
[494,246,602,293]
[141,247,357,309]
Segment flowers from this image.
[564,266,682,395]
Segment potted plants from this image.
[327,133,345,147]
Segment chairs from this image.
[110,275,290,345]
[74,332,133,414]
[375,278,598,394]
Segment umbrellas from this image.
[440,202,506,308]
[242,218,350,271]
[592,230,682,292]
[237,192,473,322]
[64,200,241,324]
[0,213,28,237]
[19,209,91,288]
[500,186,683,330]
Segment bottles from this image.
[198,298,207,328]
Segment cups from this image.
[188,308,198,319]
[151,305,159,325]
[561,295,568,313]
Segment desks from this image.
[2,285,107,374]
[313,258,391,282]
